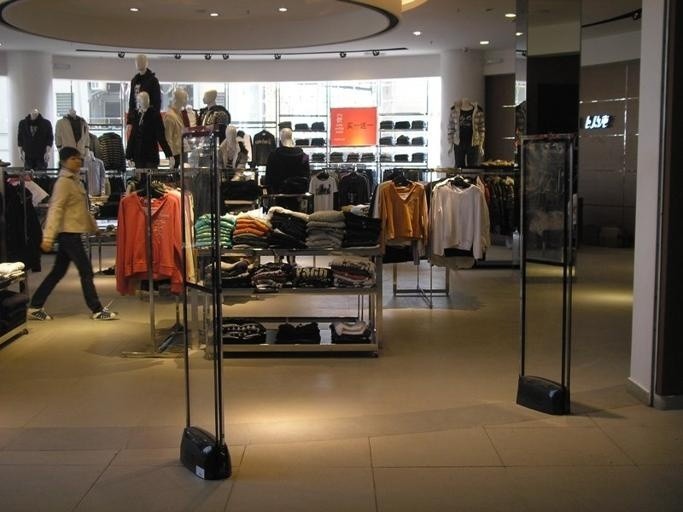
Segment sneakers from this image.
[25,306,51,321]
[92,308,117,321]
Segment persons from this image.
[196,89,230,140]
[160,88,189,164]
[214,124,249,182]
[123,54,161,142]
[444,97,489,168]
[122,91,175,175]
[180,106,201,127]
[14,108,53,170]
[24,145,119,322]
[259,127,311,267]
[50,109,89,170]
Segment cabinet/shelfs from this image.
[193,242,384,362]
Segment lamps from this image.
[205,54,211,60]
[274,53,281,60]
[118,51,125,58]
[372,50,379,56]
[339,52,346,58]
[223,54,229,60]
[174,53,181,60]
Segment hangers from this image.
[449,166,471,190]
[33,170,58,180]
[4,174,27,192]
[135,167,177,199]
[466,171,512,181]
[316,167,335,180]
[350,164,359,180]
[259,124,269,135]
[388,167,414,189]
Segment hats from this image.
[280,128,296,148]
